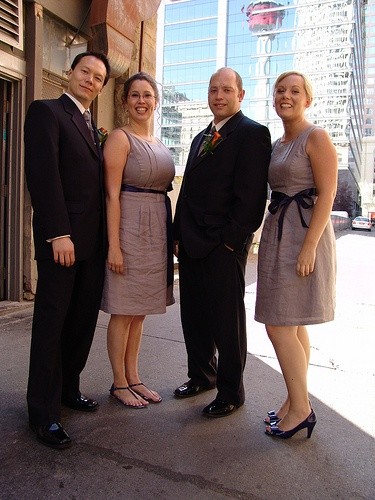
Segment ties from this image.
[83,111,96,146]
[197,126,216,159]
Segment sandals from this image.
[108,382,162,408]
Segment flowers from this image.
[203,130,221,156]
[97,127,109,145]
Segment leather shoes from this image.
[173,378,217,398]
[202,396,245,418]
[67,393,100,412]
[29,419,71,449]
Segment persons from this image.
[172,67,272,418]
[102,71,175,408]
[24,52,111,447]
[254,71,338,439]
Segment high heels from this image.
[263,400,316,438]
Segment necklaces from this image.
[131,126,153,142]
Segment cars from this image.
[352,216,372,231]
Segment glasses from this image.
[127,93,156,101]
